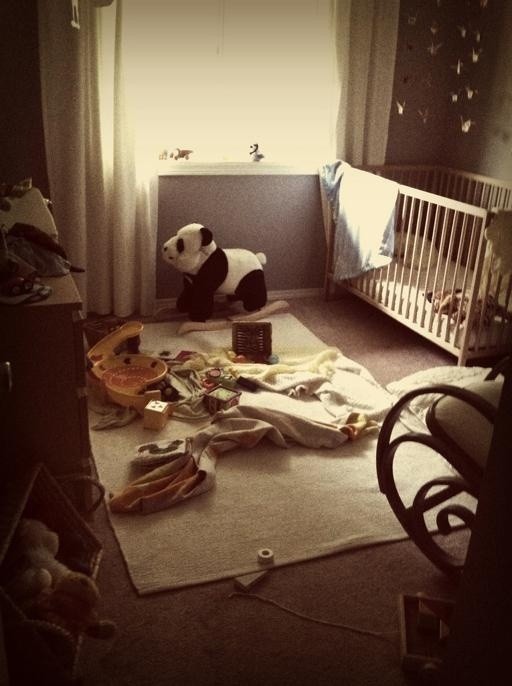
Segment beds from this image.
[318,162,512,368]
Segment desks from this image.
[0,271,92,524]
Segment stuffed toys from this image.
[12,520,116,639]
[426,286,511,328]
[159,223,267,321]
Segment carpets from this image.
[85,312,478,595]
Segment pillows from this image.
[394,231,438,268]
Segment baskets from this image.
[83,318,141,354]
[231,321,272,356]
[3,465,106,682]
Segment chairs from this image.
[376,355,511,680]
